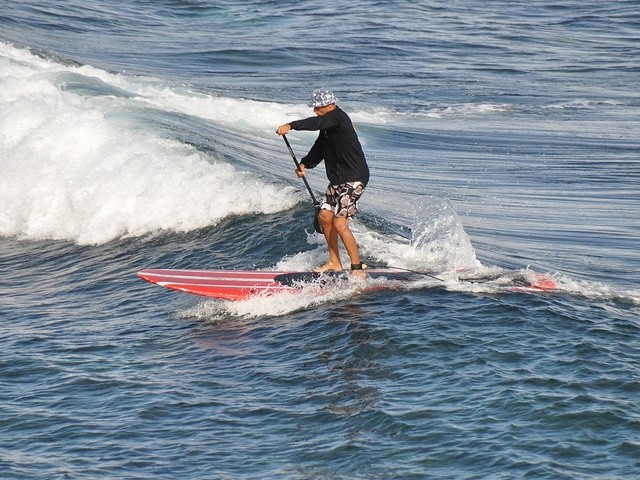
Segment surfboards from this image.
[137,268,559,301]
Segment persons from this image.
[275,86,371,281]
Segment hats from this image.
[308,87,338,107]
